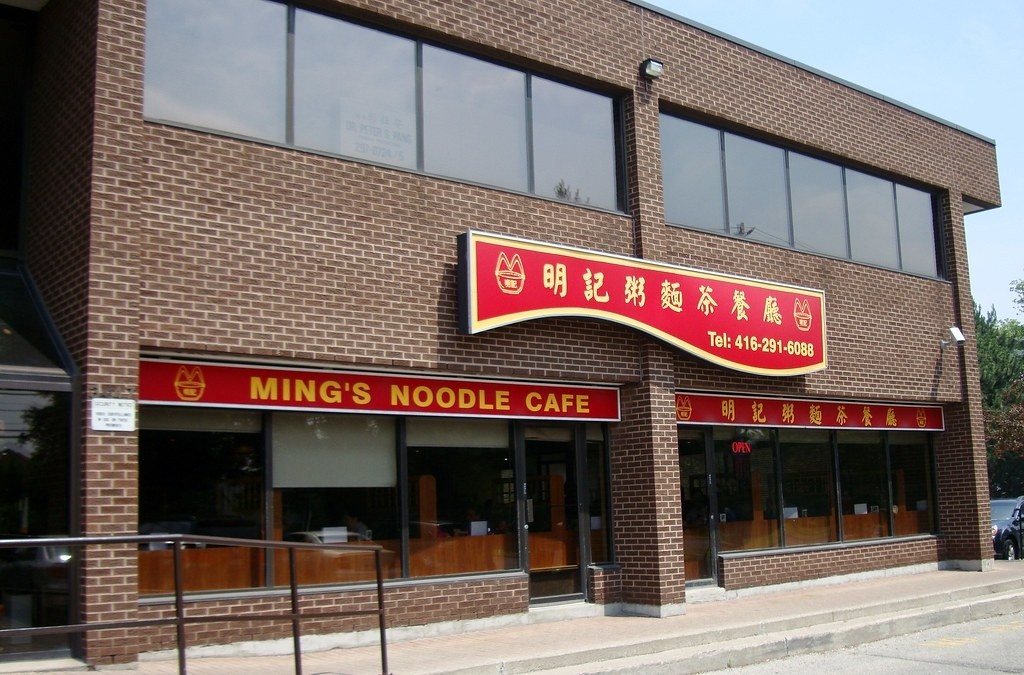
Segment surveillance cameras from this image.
[949,327,965,345]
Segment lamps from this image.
[640,57,664,81]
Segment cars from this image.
[407,518,457,539]
[286,531,375,544]
[990,495,1024,561]
[138,519,208,551]
[13,532,72,565]
